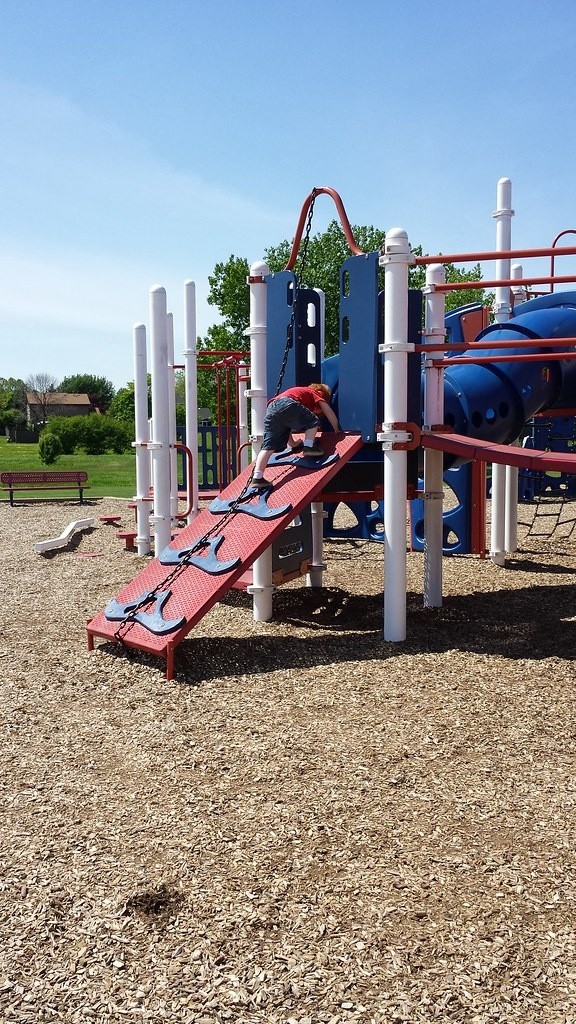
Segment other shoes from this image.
[251,478,272,487]
[303,445,325,456]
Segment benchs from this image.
[0,471,90,507]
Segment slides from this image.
[322,290,576,491]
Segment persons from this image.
[251,383,342,488]
